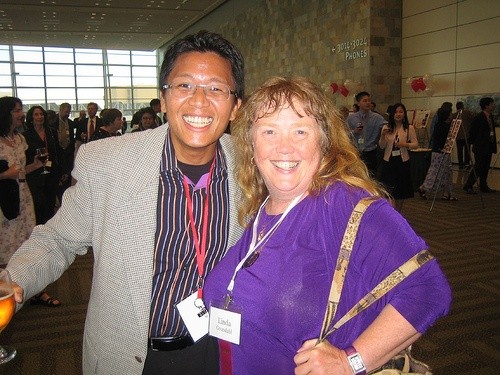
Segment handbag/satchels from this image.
[316,197,434,375]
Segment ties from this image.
[90,120,95,138]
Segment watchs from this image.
[343,345,367,375]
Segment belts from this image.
[148,336,191,351]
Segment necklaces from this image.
[243,191,306,267]
[257,192,301,241]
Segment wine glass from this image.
[0,267,17,365]
[383,124,392,145]
[356,122,365,139]
[15,156,26,181]
[35,146,51,175]
[82,129,87,142]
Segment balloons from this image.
[320,79,355,97]
[410,75,434,96]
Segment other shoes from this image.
[482,188,496,193]
[0,345,16,364]
[463,187,477,195]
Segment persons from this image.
[353,104,360,112]
[130,99,167,133]
[0,97,61,307]
[449,102,473,170]
[203,76,450,375]
[379,103,418,213]
[463,97,497,194]
[370,102,381,114]
[347,91,388,176]
[74,103,128,142]
[0,31,255,375]
[418,102,457,201]
[21,106,59,225]
[48,103,74,206]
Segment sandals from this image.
[32,292,61,307]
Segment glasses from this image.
[162,80,234,100]
